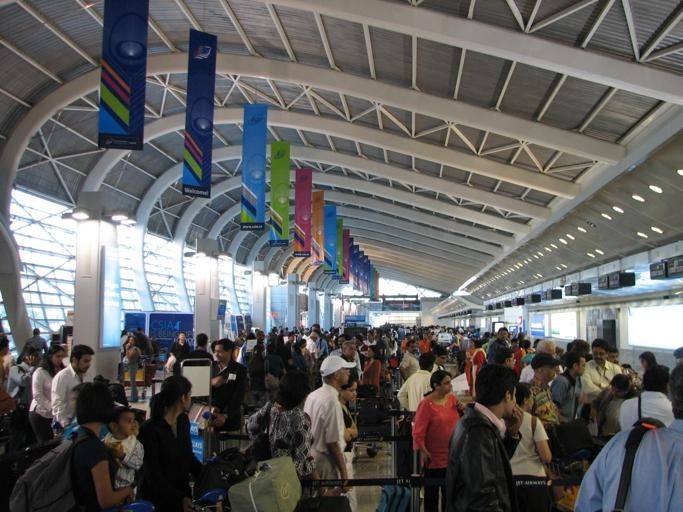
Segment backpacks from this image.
[7,429,97,511]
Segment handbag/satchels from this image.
[241,433,273,464]
[186,443,251,503]
[185,403,221,434]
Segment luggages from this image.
[374,462,429,512]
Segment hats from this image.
[530,352,563,369]
[318,354,357,378]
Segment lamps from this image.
[63,205,138,229]
[242,268,361,307]
[183,248,234,264]
